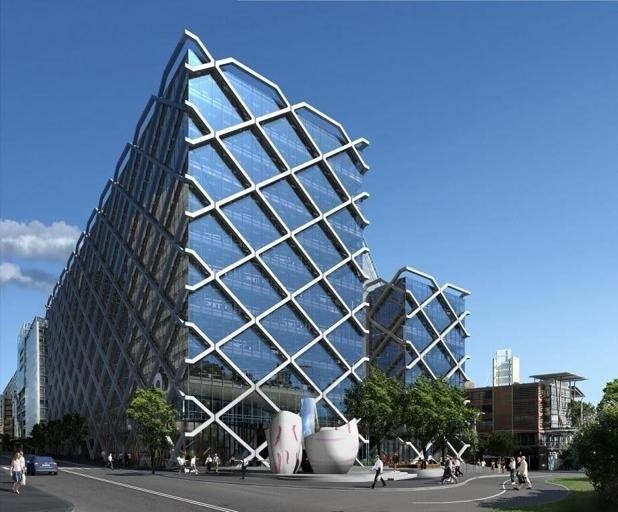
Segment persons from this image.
[100,449,131,470]
[370,455,386,489]
[380,451,399,468]
[476,455,533,490]
[175,453,221,476]
[418,458,424,468]
[240,456,248,479]
[438,453,464,485]
[10,452,22,495]
[20,450,27,489]
[230,456,235,466]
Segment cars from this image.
[27,456,58,475]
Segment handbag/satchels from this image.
[19,474,26,485]
[519,475,526,483]
